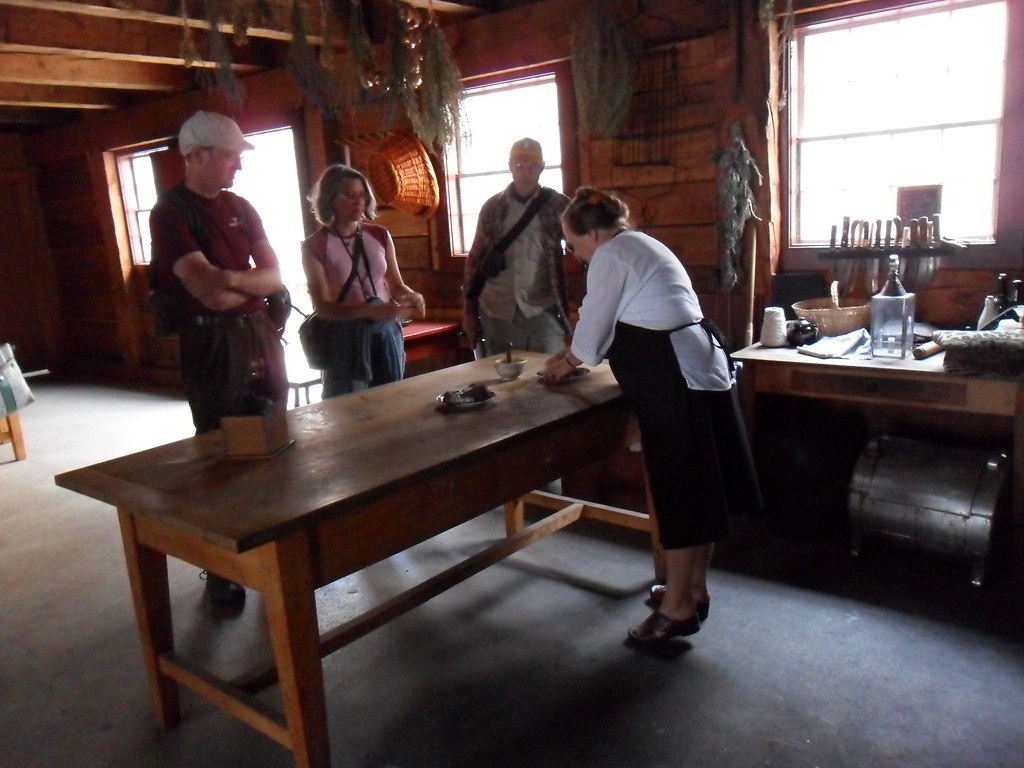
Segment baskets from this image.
[368,131,440,218]
[791,280,871,333]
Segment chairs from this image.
[272,305,323,406]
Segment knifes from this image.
[825,215,939,296]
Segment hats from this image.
[178,111,255,154]
[510,138,542,162]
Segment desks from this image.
[55,351,671,768]
[729,329,1024,550]
[392,319,459,373]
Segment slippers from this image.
[629,610,701,645]
[651,584,710,621]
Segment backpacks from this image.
[146,188,245,337]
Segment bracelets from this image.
[221,266,237,289]
[565,358,577,368]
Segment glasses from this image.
[336,194,369,205]
[511,163,539,170]
[210,148,246,162]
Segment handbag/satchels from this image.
[299,312,330,369]
[466,249,503,302]
[0,343,34,418]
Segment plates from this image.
[537,367,590,380]
[401,319,412,326]
[435,391,496,409]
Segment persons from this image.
[148,110,289,614]
[462,137,571,360]
[299,163,426,400]
[545,180,762,643]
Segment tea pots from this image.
[788,317,818,346]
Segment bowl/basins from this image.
[494,358,527,380]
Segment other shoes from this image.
[200,569,245,606]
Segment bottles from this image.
[870,255,916,359]
[1010,279,1024,308]
[994,272,1009,312]
[976,295,997,331]
